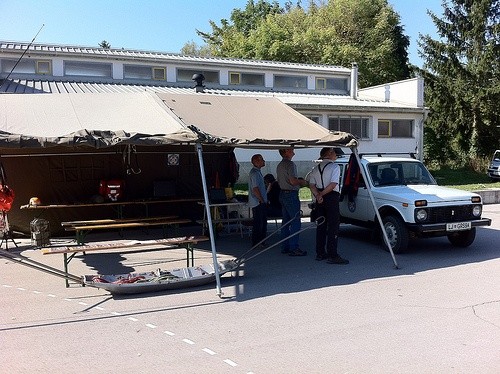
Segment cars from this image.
[488,150,500,182]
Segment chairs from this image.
[381,168,396,185]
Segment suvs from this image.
[333,151,492,256]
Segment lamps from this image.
[167,154,180,166]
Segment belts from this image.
[280,189,296,192]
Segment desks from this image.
[20,197,247,243]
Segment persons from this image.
[309,147,349,265]
[262,174,283,219]
[247,154,271,250]
[276,147,308,256]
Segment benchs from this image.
[40,234,209,288]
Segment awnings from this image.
[0,90,402,298]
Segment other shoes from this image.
[288,249,307,256]
[316,254,327,260]
[281,248,288,253]
[327,255,349,264]
